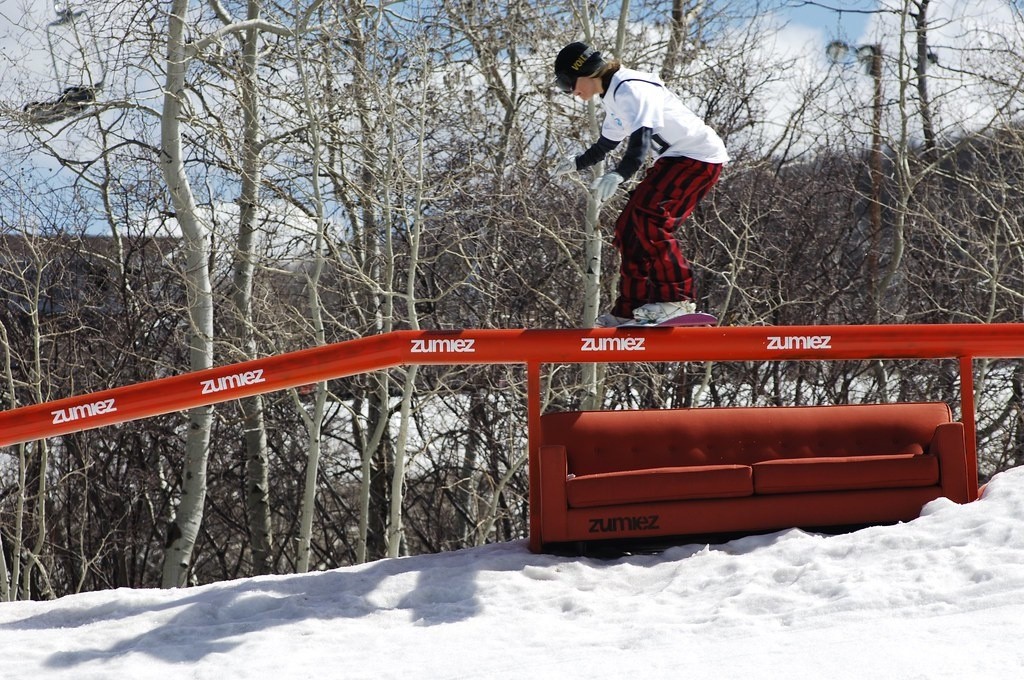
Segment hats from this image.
[554,42,603,90]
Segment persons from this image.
[554,41,731,328]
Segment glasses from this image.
[554,74,573,95]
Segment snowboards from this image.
[595,311,719,328]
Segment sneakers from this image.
[632,300,697,323]
[594,314,631,327]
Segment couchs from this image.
[537,402,969,546]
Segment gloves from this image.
[590,171,625,201]
[550,155,577,178]
